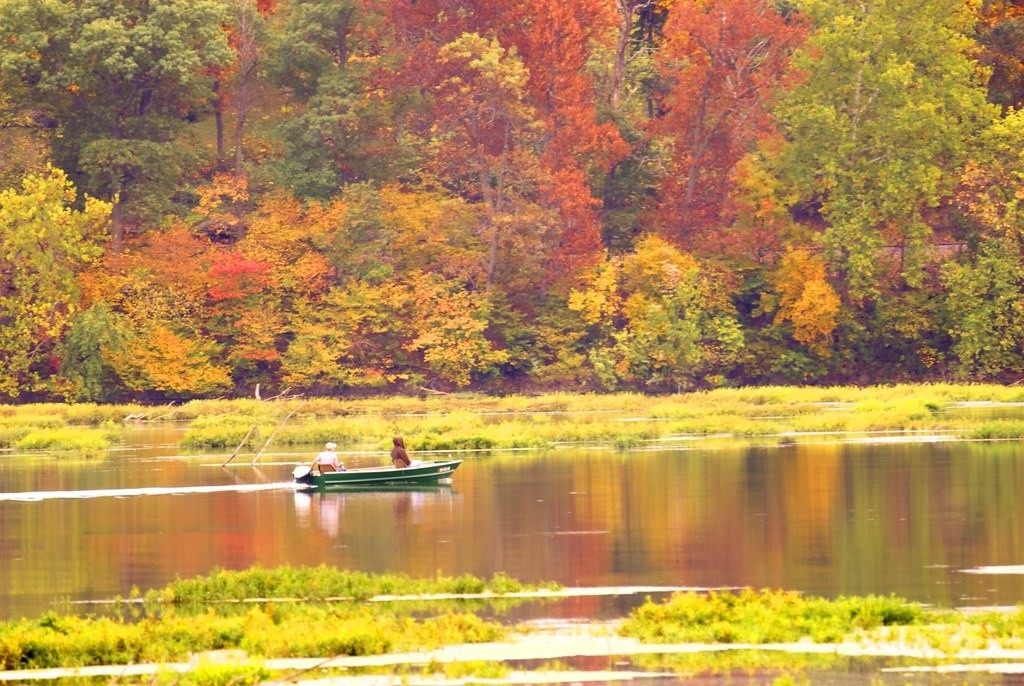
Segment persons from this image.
[389,434,423,467]
[309,442,346,475]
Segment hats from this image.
[327,442,337,449]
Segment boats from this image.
[295,458,463,482]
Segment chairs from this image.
[317,464,337,474]
[393,459,408,468]
